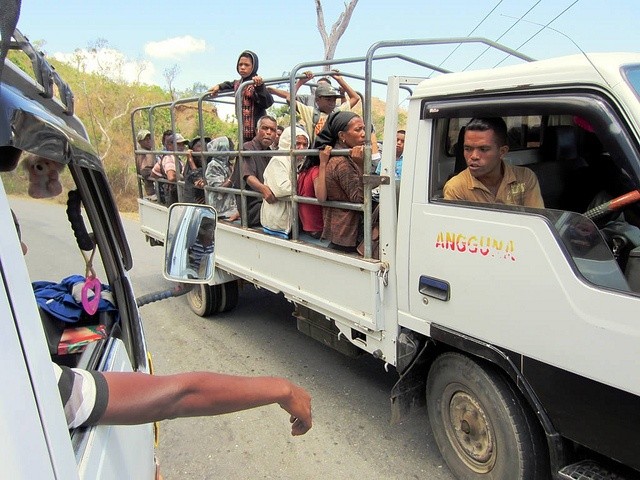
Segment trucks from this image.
[0,0,218,480]
[129,36,640,480]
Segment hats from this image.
[169,133,188,143]
[136,129,151,144]
[190,134,211,145]
[315,85,344,101]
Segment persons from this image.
[205,51,274,146]
[259,126,310,235]
[266,78,348,108]
[296,111,379,252]
[268,126,285,150]
[442,112,545,209]
[204,136,237,218]
[8,206,313,436]
[326,246,329,253]
[150,133,191,181]
[154,130,171,160]
[183,134,210,204]
[238,114,275,226]
[286,69,360,139]
[295,144,332,232]
[136,129,158,200]
[187,218,214,271]
[374,129,406,204]
